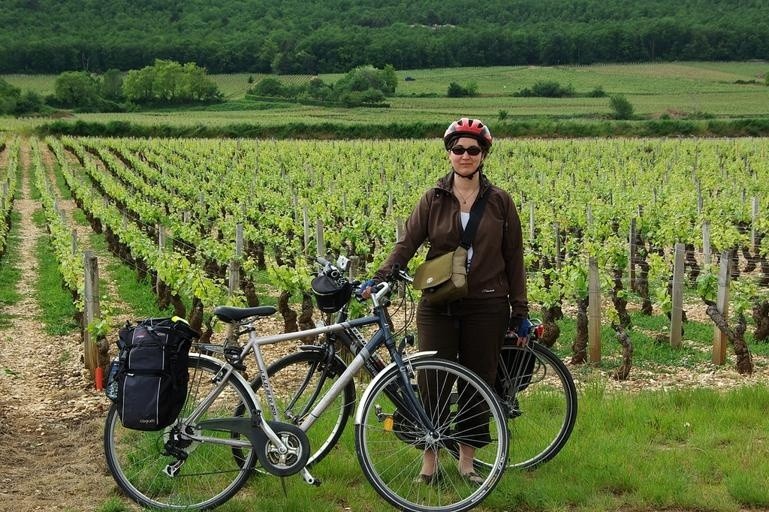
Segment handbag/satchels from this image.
[412,247,469,308]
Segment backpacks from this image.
[116,316,200,431]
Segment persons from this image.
[354,118,533,487]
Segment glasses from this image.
[451,145,482,156]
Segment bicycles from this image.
[101,283,508,511]
[230,251,579,477]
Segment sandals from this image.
[415,470,442,486]
[461,471,483,486]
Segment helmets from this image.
[444,118,492,150]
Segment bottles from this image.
[106,355,122,400]
